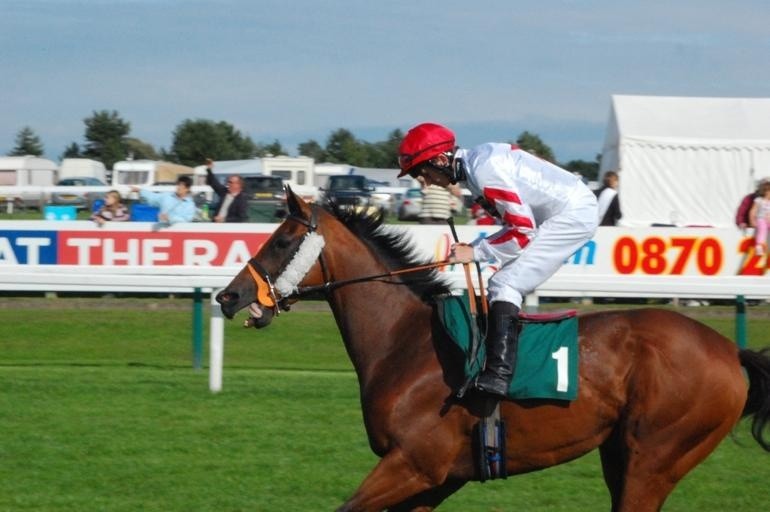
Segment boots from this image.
[468,302,522,396]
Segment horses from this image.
[213,184,769,512]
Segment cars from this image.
[145,181,213,207]
[51,177,133,208]
[398,188,424,222]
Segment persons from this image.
[392,119,600,402]
[88,189,132,227]
[735,178,770,230]
[746,180,770,258]
[127,174,197,226]
[201,156,250,223]
[591,169,622,227]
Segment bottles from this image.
[202,204,209,222]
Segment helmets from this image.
[397,123,455,178]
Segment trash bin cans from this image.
[130,202,160,222]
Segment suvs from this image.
[317,175,375,208]
[242,175,289,218]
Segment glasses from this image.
[398,153,413,168]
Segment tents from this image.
[597,93,770,229]
